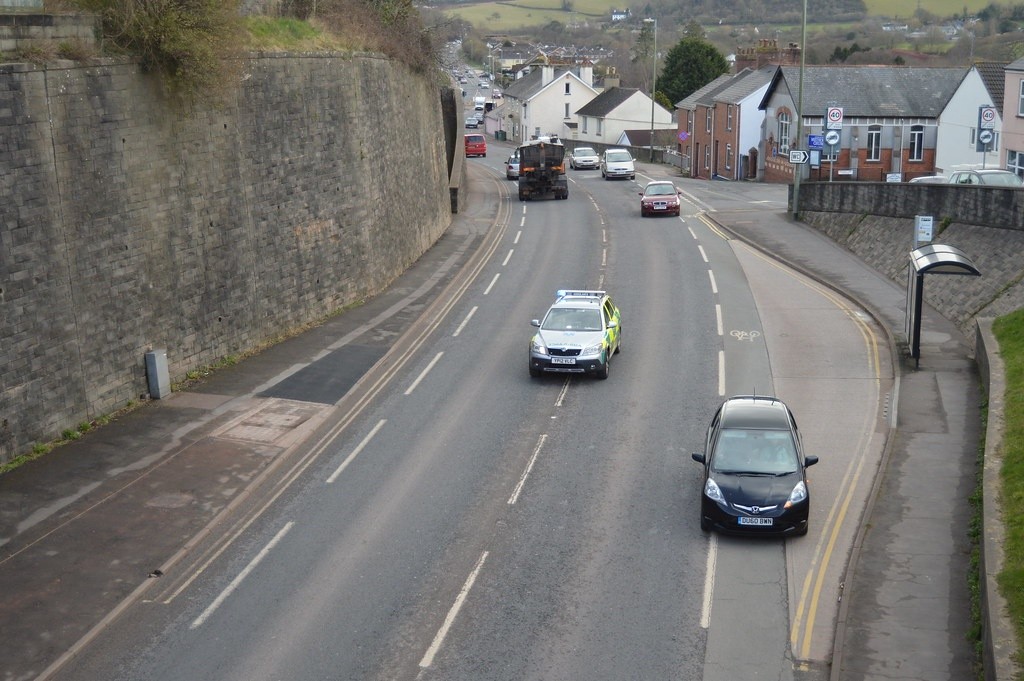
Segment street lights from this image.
[643,18,657,163]
[483,62,485,73]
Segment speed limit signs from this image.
[829,109,842,121]
[983,109,994,122]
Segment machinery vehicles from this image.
[517,134,569,201]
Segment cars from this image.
[460,78,468,84]
[468,72,475,78]
[478,80,484,86]
[464,133,487,158]
[492,89,502,99]
[691,394,819,540]
[947,170,1022,187]
[478,73,488,77]
[908,175,948,183]
[505,155,520,180]
[445,36,461,54]
[600,148,637,181]
[528,289,622,379]
[569,147,600,170]
[481,82,489,89]
[638,180,682,218]
[458,75,464,81]
[455,71,461,77]
[472,113,483,124]
[453,67,458,74]
[465,118,479,128]
[464,66,471,73]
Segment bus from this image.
[882,22,909,32]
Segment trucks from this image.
[475,96,485,110]
